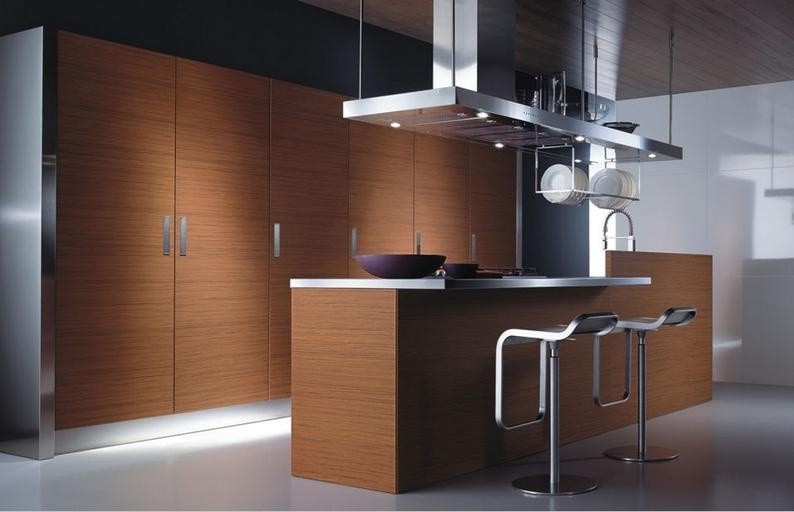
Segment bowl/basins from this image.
[601,119,642,135]
[566,97,611,121]
[440,262,480,279]
[350,253,448,279]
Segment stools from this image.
[487,307,622,500]
[599,304,698,466]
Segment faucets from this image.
[602,210,635,252]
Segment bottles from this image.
[524,72,543,110]
[546,68,566,117]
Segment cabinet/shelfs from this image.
[0,23,269,465]
[268,76,349,405]
[349,96,414,278]
[468,139,516,270]
[415,131,468,265]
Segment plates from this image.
[539,164,637,213]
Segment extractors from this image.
[341,0,683,169]
